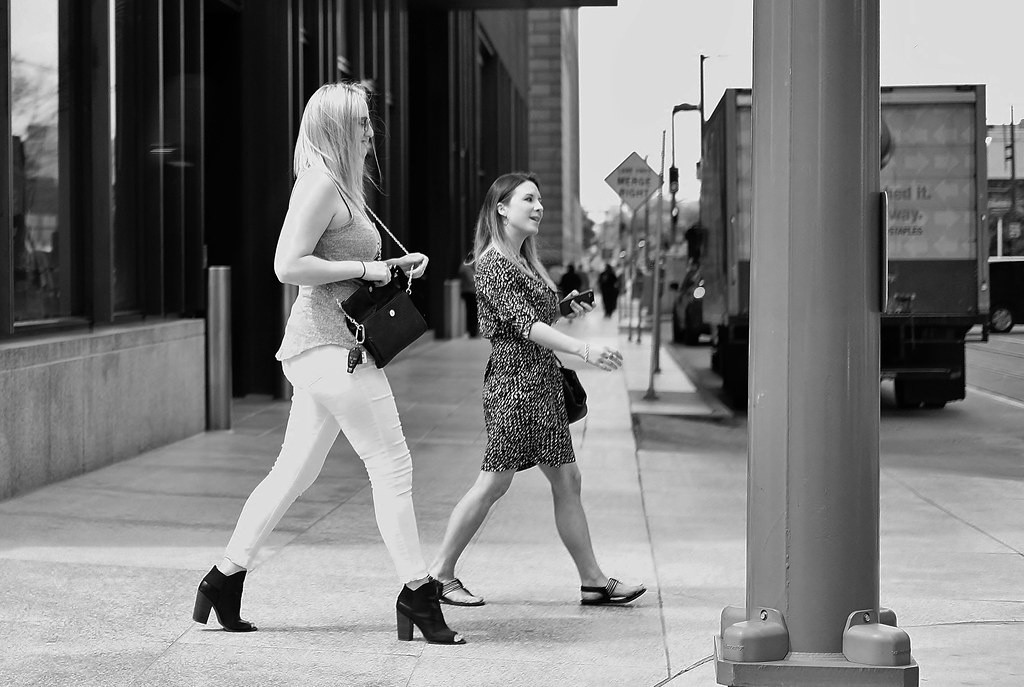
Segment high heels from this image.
[395,577,466,645]
[192,565,258,632]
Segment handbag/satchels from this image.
[558,366,589,425]
[340,264,429,369]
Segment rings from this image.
[609,352,612,358]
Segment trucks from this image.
[686,83,986,411]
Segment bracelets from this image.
[583,343,590,365]
[354,259,366,280]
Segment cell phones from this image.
[559,289,595,316]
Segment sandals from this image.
[578,578,647,606]
[436,579,485,606]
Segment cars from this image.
[669,272,709,345]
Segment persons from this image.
[427,170,647,607]
[193,80,467,645]
[553,259,626,319]
